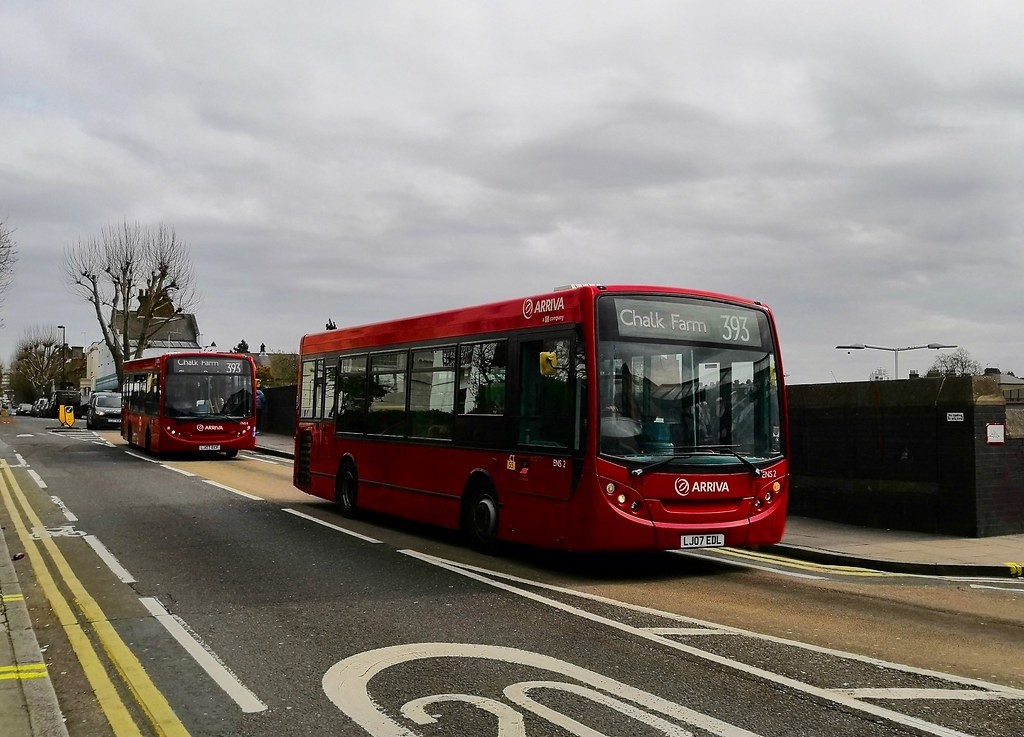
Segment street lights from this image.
[57,325,66,390]
[835,343,958,380]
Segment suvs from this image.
[87,392,122,430]
[47,390,82,419]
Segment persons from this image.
[568,367,618,428]
[256,378,266,434]
[147,386,155,398]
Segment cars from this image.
[2,398,49,418]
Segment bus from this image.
[291,283,790,568]
[121,351,257,460]
